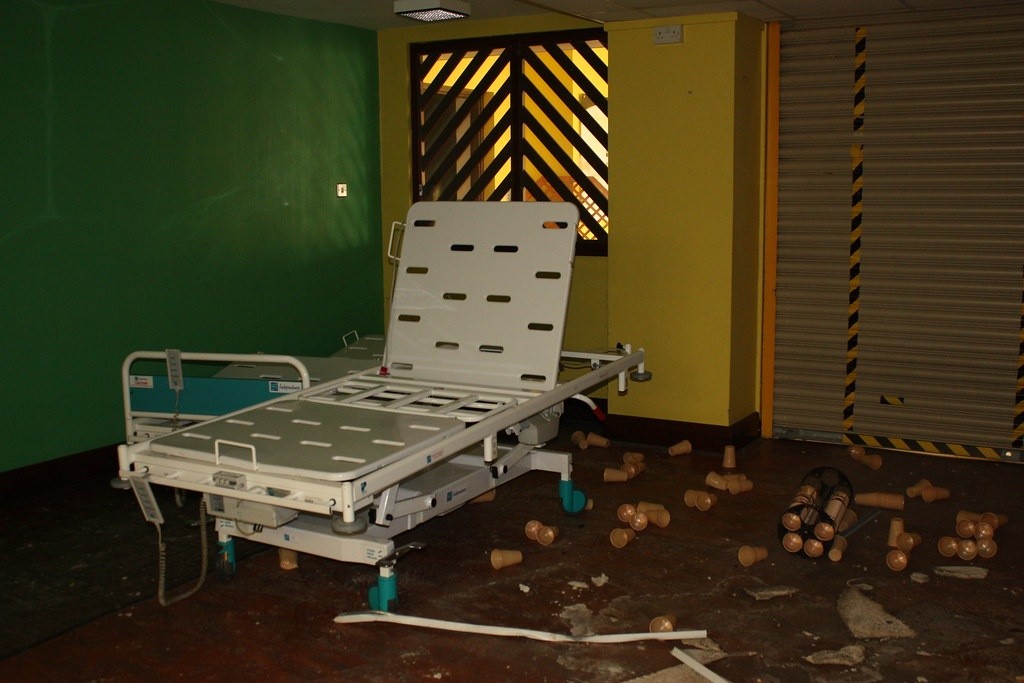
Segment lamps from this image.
[394,0,471,25]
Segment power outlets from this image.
[654,23,683,45]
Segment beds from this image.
[118,201,654,613]
